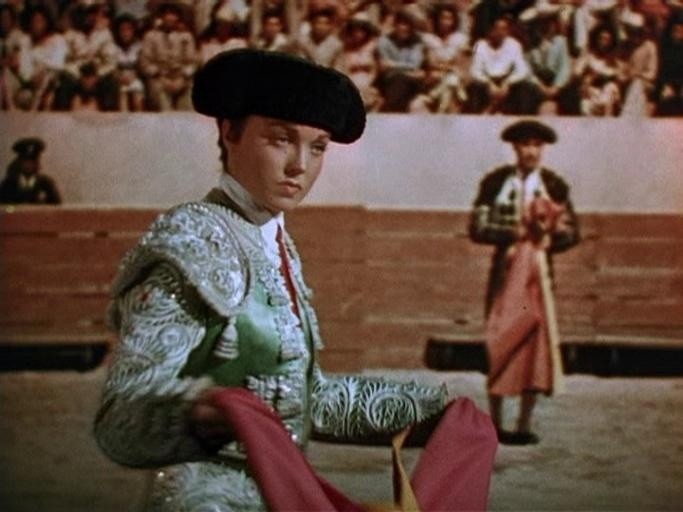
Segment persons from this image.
[88,46,462,511]
[0,136,63,205]
[1,0,683,120]
[465,118,582,446]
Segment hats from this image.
[519,0,564,21]
[347,11,383,39]
[500,120,557,146]
[190,46,366,145]
[617,12,645,28]
[395,5,429,33]
[12,137,47,155]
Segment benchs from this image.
[0,333,108,370]
[425,333,683,380]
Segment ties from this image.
[276,224,300,325]
